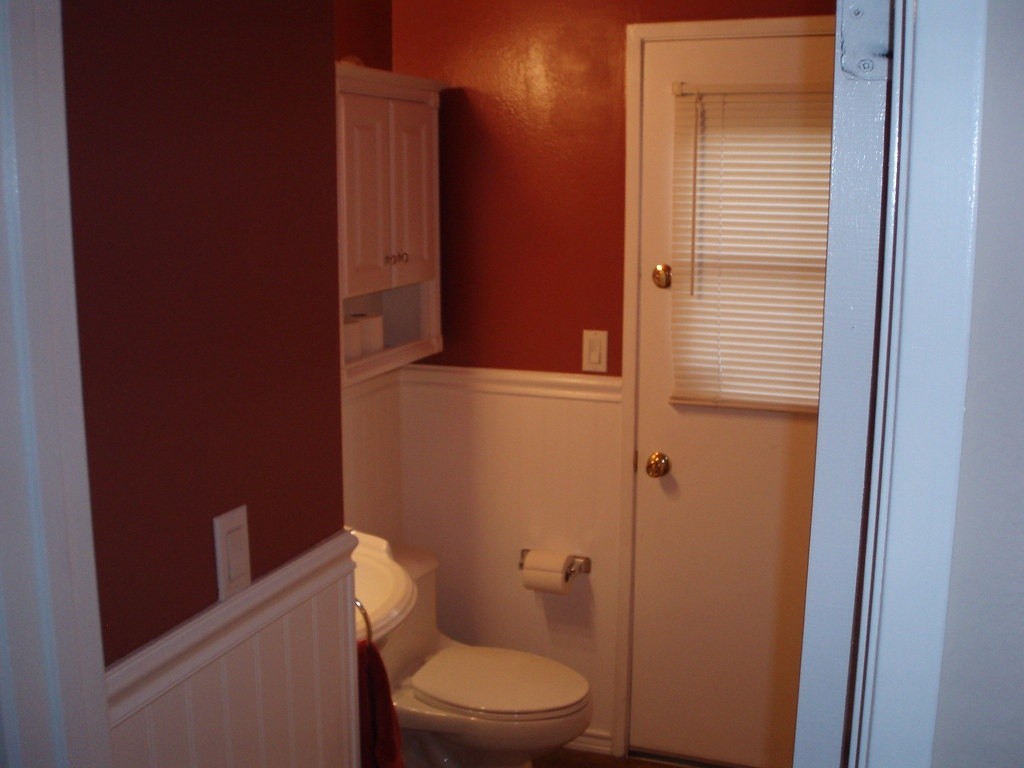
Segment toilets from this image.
[360,538,596,753]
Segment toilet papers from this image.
[522,547,576,596]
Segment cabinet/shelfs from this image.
[337,62,442,389]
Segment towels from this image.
[357,639,412,768]
